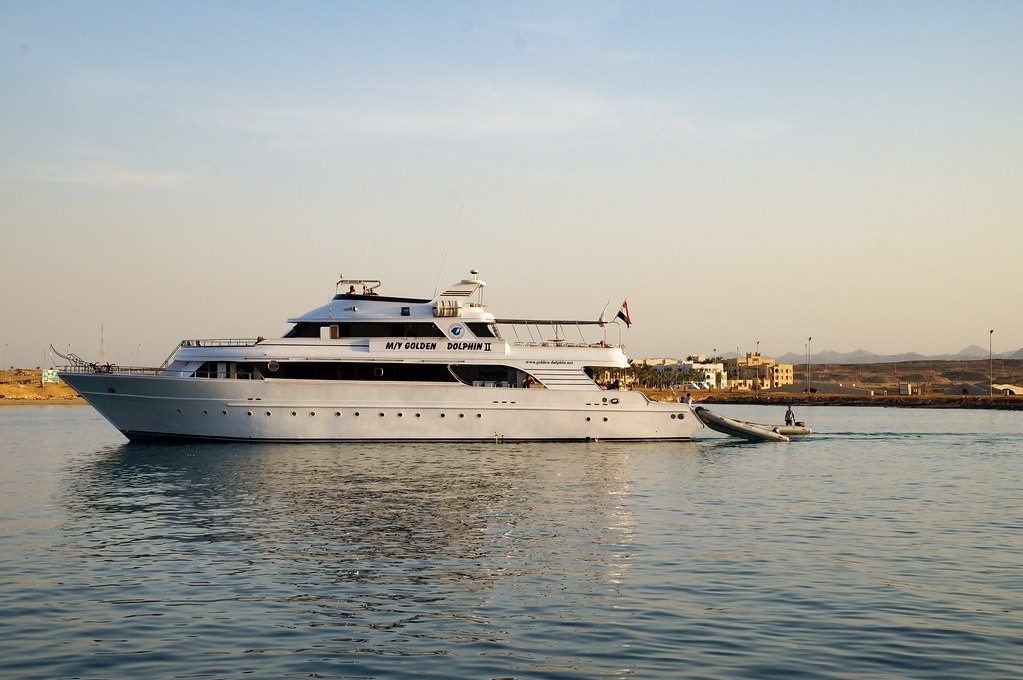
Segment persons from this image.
[709,388,712,393]
[96,366,105,373]
[595,373,620,390]
[346,285,379,295]
[521,374,535,388]
[785,405,795,426]
[680,393,696,404]
[196,340,201,346]
[653,386,655,390]
[187,341,190,347]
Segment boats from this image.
[745,421,812,436]
[695,405,790,444]
[46,252,708,445]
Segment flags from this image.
[617,300,632,328]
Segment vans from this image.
[673,380,709,390]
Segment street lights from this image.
[808,337,812,393]
[136,343,142,366]
[756,341,760,395]
[989,329,994,397]
